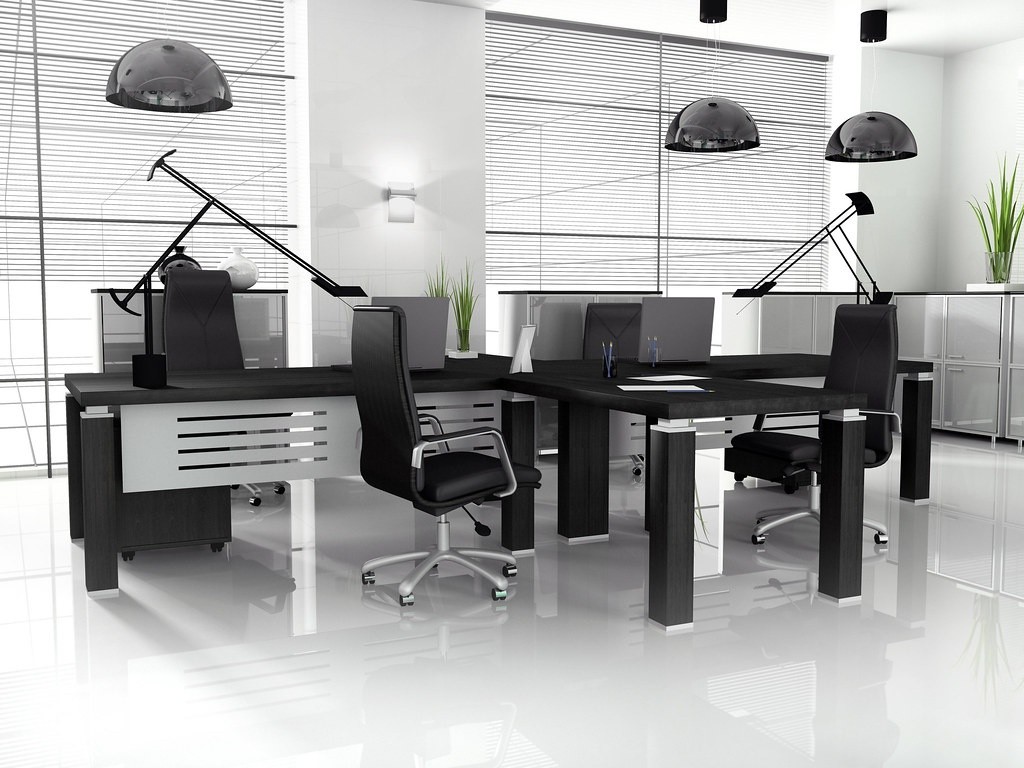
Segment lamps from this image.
[105,0,233,114]
[825,11,918,163]
[92,149,367,390]
[733,191,894,305]
[664,0,761,153]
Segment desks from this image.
[66,354,935,633]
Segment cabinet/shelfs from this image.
[721,293,1024,448]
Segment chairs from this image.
[161,267,286,506]
[350,306,545,611]
[731,305,901,546]
[582,303,644,476]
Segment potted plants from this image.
[965,149,1024,285]
[961,582,1016,704]
[448,258,482,352]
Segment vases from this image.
[218,246,259,290]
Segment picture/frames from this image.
[509,325,537,374]
